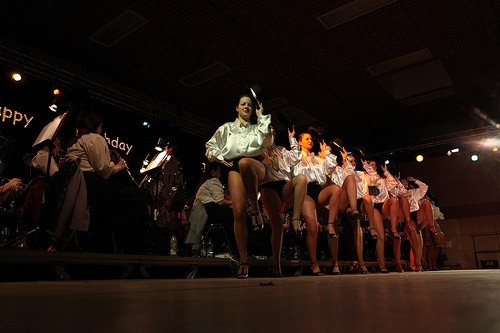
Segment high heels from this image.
[250,209,264,231]
[348,208,438,242]
[237,259,250,278]
[272,263,283,277]
[307,265,439,276]
[290,217,302,236]
[324,221,338,239]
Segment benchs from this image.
[440,263,460,270]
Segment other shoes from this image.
[215,252,234,259]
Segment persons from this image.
[27,93,445,278]
[0,178,22,204]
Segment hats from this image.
[347,143,367,161]
[390,161,400,177]
[371,152,388,167]
[330,137,344,152]
[243,82,265,110]
[308,126,327,142]
[275,105,294,131]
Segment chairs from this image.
[194,198,233,258]
[16,157,118,253]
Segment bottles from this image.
[199,240,206,258]
[170,234,177,255]
[206,237,215,258]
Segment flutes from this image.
[101,134,142,192]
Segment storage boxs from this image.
[480,259,498,269]
